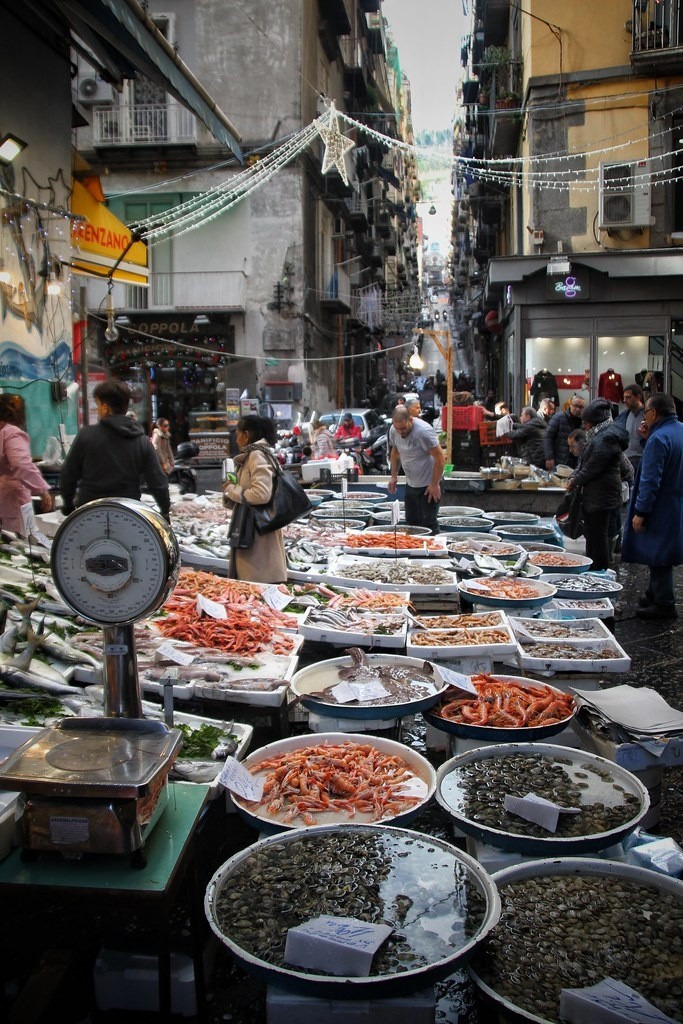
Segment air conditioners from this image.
[76,72,116,103]
[597,160,652,226]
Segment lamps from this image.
[0,132,28,166]
[116,315,131,324]
[429,206,436,215]
[194,315,211,324]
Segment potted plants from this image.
[480,88,490,104]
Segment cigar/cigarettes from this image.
[641,421,646,423]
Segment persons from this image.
[405,399,420,417]
[566,397,635,571]
[125,411,139,421]
[387,405,446,537]
[153,417,175,472]
[530,367,560,413]
[0,393,52,533]
[634,368,651,404]
[397,396,406,404]
[505,395,586,470]
[371,370,521,459]
[597,368,624,420]
[310,420,340,459]
[335,413,362,439]
[57,380,171,525]
[621,393,683,619]
[222,418,288,583]
[614,384,647,476]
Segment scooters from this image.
[360,431,391,471]
[169,442,199,494]
[444,314,447,320]
[434,314,440,321]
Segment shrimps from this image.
[153,568,299,658]
[430,671,578,728]
[467,578,539,599]
[243,740,423,827]
[334,559,451,584]
[347,532,443,550]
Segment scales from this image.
[0,496,183,870]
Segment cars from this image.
[320,406,388,457]
[402,307,430,390]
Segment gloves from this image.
[159,510,171,526]
[60,503,75,516]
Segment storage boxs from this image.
[570,718,683,771]
[478,421,513,445]
[91,947,198,1016]
[444,404,484,431]
[266,982,437,1024]
[302,462,332,482]
[177,443,199,458]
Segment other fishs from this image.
[210,720,240,761]
[0,530,266,718]
[171,498,350,572]
[211,678,291,692]
[412,612,508,647]
[172,760,227,784]
[549,574,620,592]
[558,599,610,610]
[291,584,416,635]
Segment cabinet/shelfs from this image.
[187,411,231,467]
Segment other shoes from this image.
[637,595,657,606]
[636,604,677,620]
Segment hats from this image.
[579,396,612,422]
[343,413,352,420]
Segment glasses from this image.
[644,408,653,415]
[159,424,170,427]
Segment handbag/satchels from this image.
[556,483,586,540]
[227,489,256,550]
[250,445,314,537]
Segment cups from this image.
[347,468,359,482]
[320,468,331,483]
